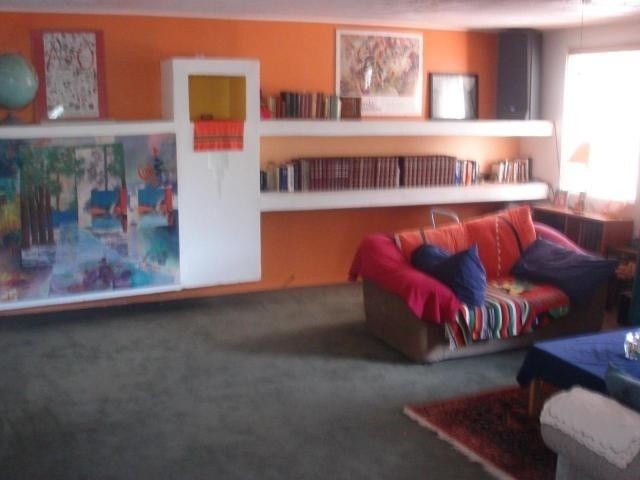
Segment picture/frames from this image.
[31,27,106,124]
[429,73,479,120]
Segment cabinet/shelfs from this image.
[259,119,554,212]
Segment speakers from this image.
[496,29,543,121]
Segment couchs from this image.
[359,223,607,364]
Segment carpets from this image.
[402,384,558,480]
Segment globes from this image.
[0,54,39,126]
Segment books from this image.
[603,251,638,326]
[260,153,532,193]
[191,117,244,152]
[260,89,363,121]
[533,211,604,251]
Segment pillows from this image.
[393,204,610,306]
